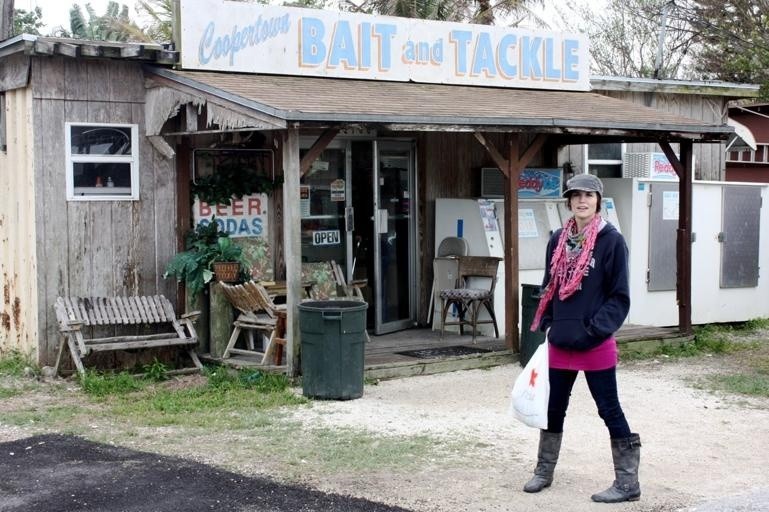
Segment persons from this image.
[521,172,642,504]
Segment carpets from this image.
[393,345,495,359]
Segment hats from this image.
[561,173,604,199]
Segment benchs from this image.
[50,293,209,388]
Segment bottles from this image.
[94,176,104,187]
[107,175,114,187]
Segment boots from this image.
[591,433,641,503]
[524,429,562,492]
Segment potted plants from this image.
[161,234,253,307]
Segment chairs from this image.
[216,276,286,366]
[426,236,470,336]
[437,254,504,345]
[301,259,372,344]
